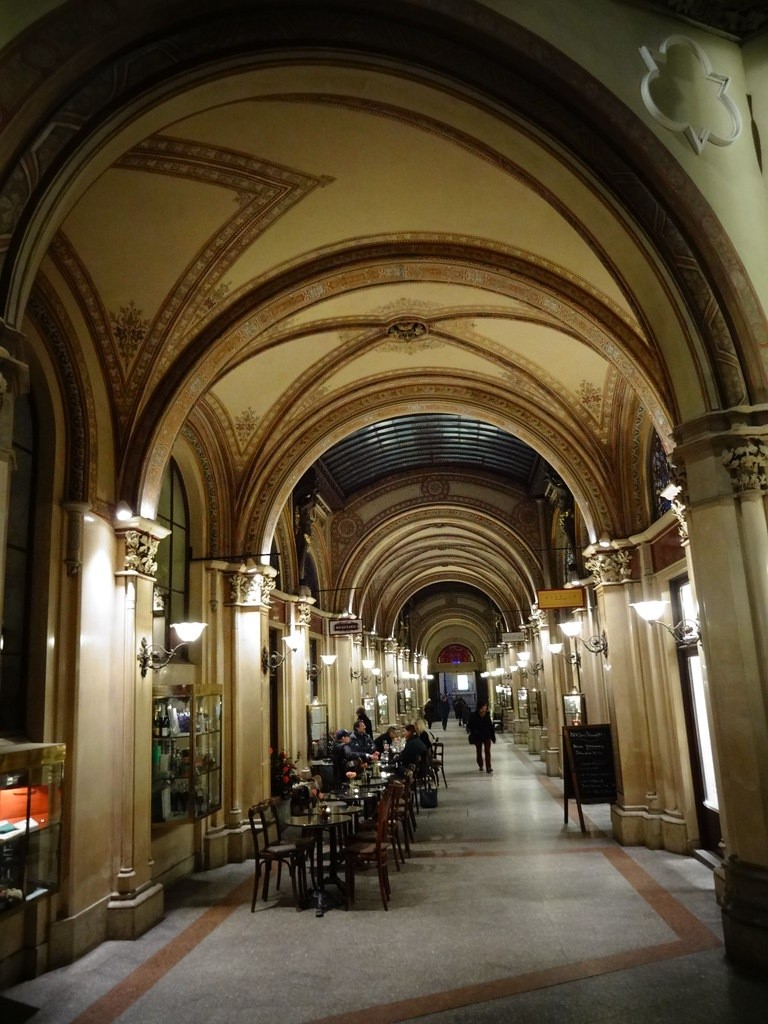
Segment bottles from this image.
[153,705,161,738]
[160,704,169,738]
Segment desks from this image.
[283,749,401,916]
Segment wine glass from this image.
[384,744,389,753]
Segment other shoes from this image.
[479,766,483,770]
[487,769,493,773]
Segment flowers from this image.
[269,749,304,797]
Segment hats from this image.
[337,729,352,738]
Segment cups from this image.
[320,801,328,812]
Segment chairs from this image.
[290,786,340,866]
[249,798,307,910]
[337,738,448,912]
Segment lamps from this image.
[510,652,544,677]
[137,621,208,676]
[262,632,307,674]
[305,654,341,683]
[559,622,608,658]
[480,667,513,681]
[376,670,393,686]
[350,659,377,682]
[546,642,581,667]
[628,601,704,650]
[361,669,381,685]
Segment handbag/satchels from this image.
[419,781,438,808]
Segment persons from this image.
[331,730,374,784]
[350,719,379,765]
[452,695,466,726]
[290,775,323,817]
[411,719,433,755]
[397,725,426,779]
[424,699,434,729]
[437,697,450,730]
[373,727,397,752]
[466,700,496,773]
[357,707,373,740]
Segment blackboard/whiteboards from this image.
[562,723,618,804]
